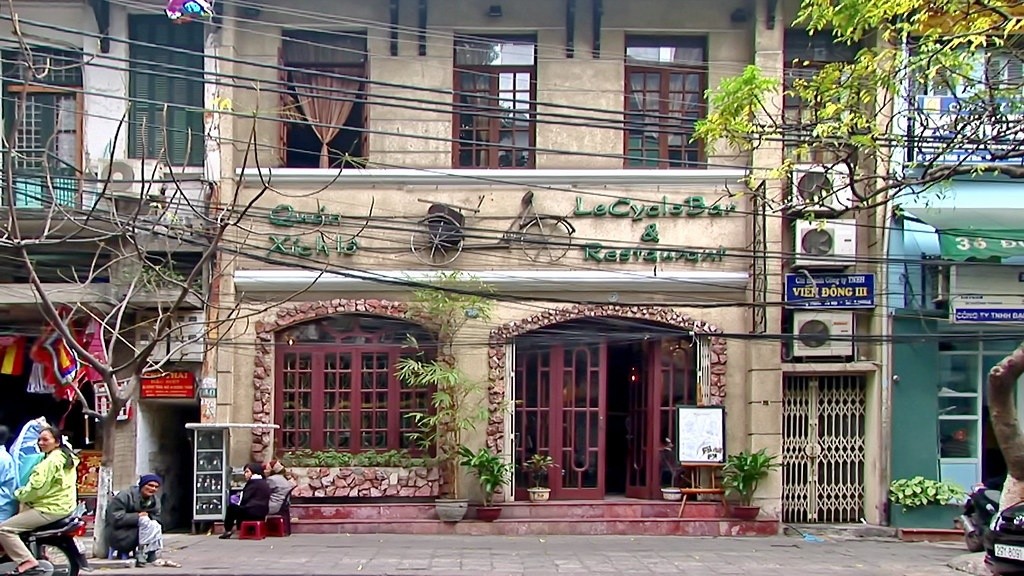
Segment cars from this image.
[984,500,1024,576]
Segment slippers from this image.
[4,567,39,575]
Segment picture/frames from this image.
[675,405,726,462]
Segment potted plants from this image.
[393,263,488,524]
[523,453,560,502]
[658,439,687,502]
[888,476,967,529]
[720,448,787,519]
[455,444,513,522]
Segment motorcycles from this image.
[0,514,88,576]
[958,482,1002,553]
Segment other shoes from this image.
[219,531,233,539]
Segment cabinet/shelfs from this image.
[192,429,228,519]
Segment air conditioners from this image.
[97,158,163,196]
[791,163,852,211]
[791,218,856,270]
[790,312,853,356]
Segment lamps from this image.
[731,7,747,23]
[243,4,262,18]
[489,6,503,16]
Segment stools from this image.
[239,521,266,539]
[109,547,135,560]
[266,515,287,537]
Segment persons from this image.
[0,428,81,576]
[218,462,295,540]
[105,474,162,568]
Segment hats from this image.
[138,475,161,488]
